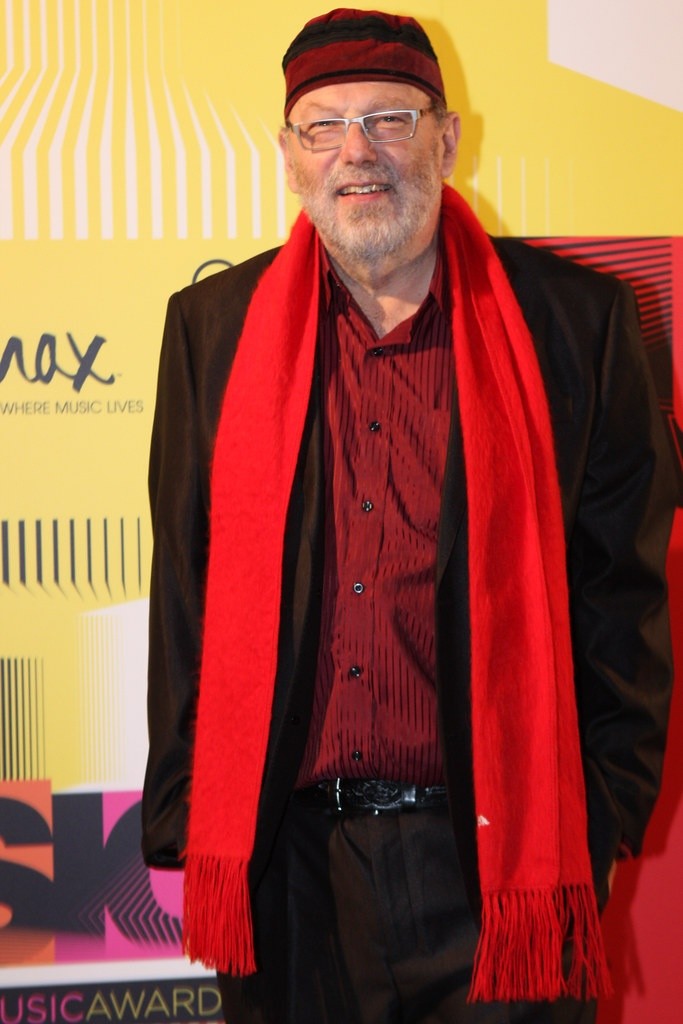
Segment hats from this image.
[283,8,447,114]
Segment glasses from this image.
[289,106,437,150]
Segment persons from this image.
[141,8,675,1024]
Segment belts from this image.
[294,779,449,821]
